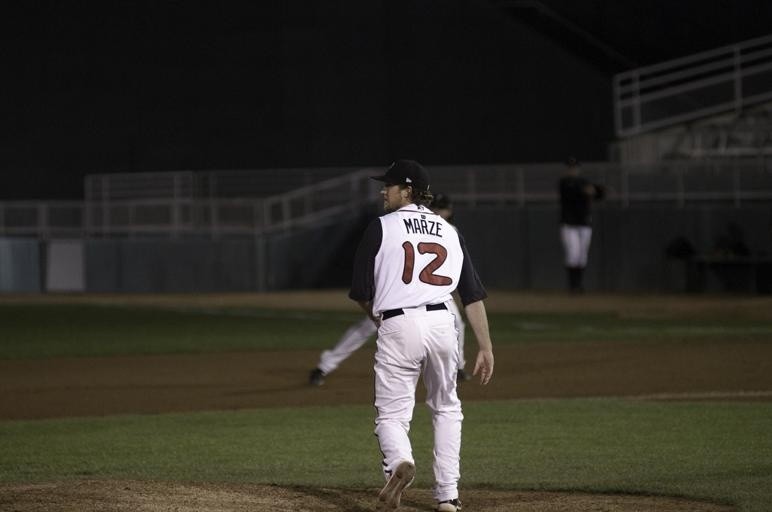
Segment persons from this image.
[309,192,474,389]
[556,155,607,297]
[349,158,495,509]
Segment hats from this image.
[427,192,452,209]
[369,159,431,192]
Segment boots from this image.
[566,263,586,295]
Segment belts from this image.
[382,303,448,321]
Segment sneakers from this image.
[437,498,463,512]
[308,368,326,389]
[378,461,416,509]
[456,369,473,385]
